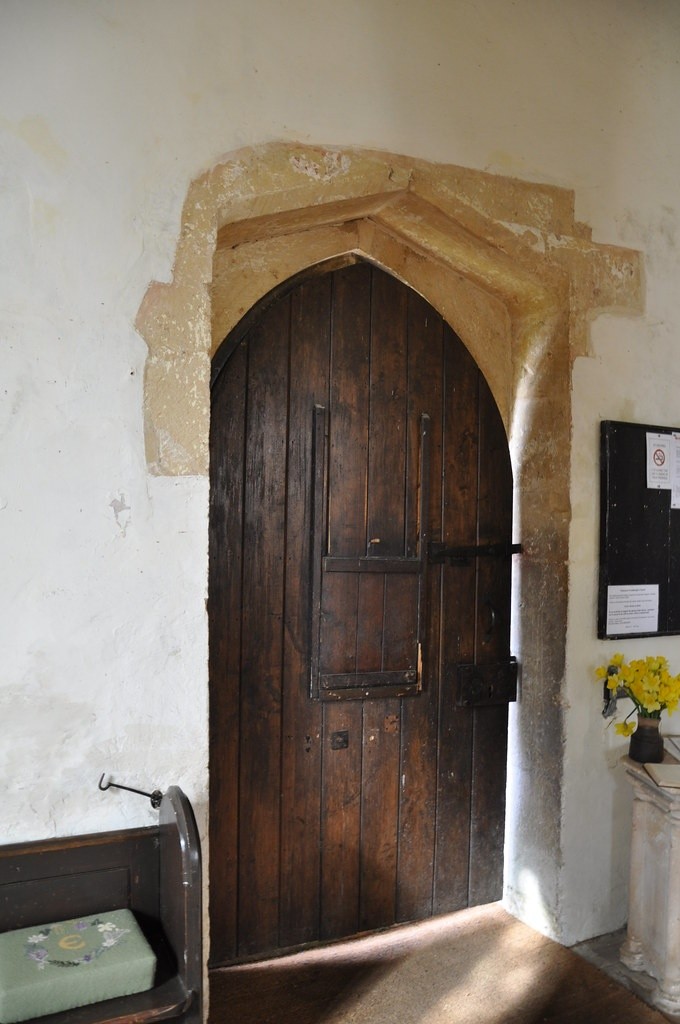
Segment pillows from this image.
[0,908,158,1024]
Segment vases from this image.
[628,715,665,764]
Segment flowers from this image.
[595,651,680,738]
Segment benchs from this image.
[0,785,203,1024]
